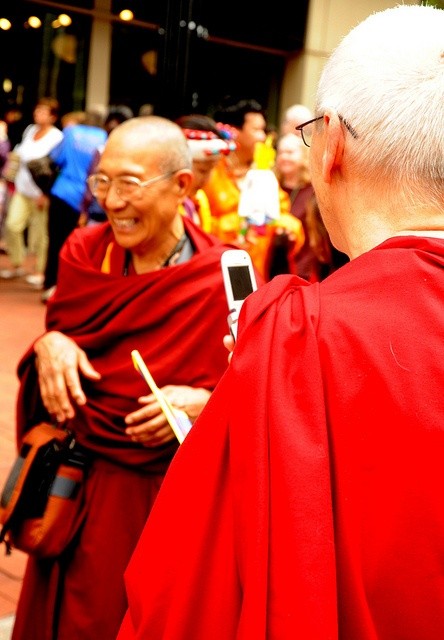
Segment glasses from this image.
[85,170,175,199]
[294,113,356,147]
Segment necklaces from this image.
[119,237,187,281]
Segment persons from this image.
[105,105,133,136]
[281,104,313,138]
[177,114,241,248]
[275,133,332,282]
[0,98,22,219]
[0,97,63,287]
[203,100,305,283]
[12,116,265,640]
[61,111,85,129]
[117,4,443,639]
[40,104,108,301]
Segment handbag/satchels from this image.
[0,418,91,560]
[28,156,56,193]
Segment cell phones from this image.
[219,250,258,343]
